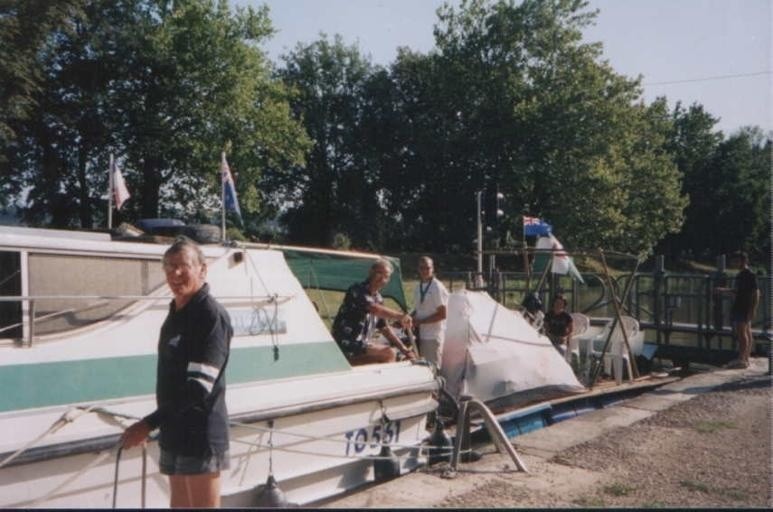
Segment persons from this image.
[489,286,575,360]
[116,240,234,509]
[713,250,760,369]
[331,258,413,366]
[408,254,448,370]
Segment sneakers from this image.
[726,359,751,369]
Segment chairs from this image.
[557,313,640,384]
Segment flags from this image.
[535,236,585,284]
[113,162,131,212]
[222,159,243,227]
[524,216,551,235]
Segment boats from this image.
[1,152,685,512]
